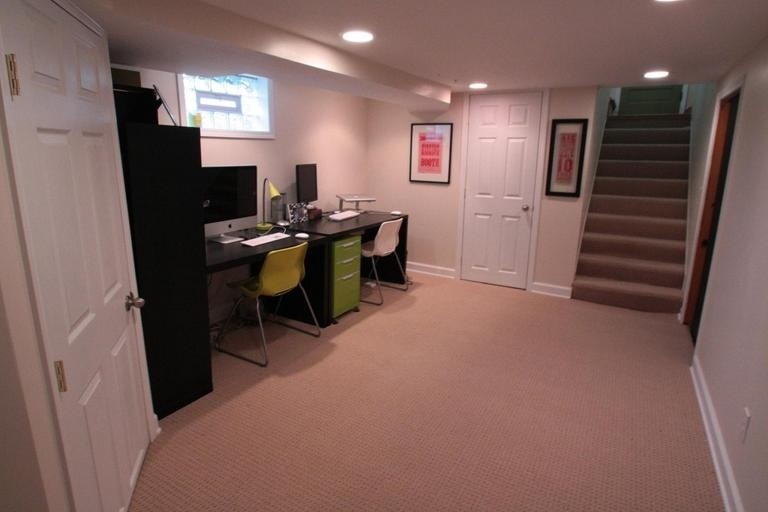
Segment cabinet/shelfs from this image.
[330,235,362,325]
[125,125,212,419]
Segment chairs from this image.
[360,218,408,305]
[214,242,321,367]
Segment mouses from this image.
[390,210,402,216]
[295,233,309,239]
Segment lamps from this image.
[256,177,282,231]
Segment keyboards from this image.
[328,210,361,221]
[240,232,291,247]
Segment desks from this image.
[272,208,408,326]
[206,226,328,328]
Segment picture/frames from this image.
[545,118,588,198]
[409,123,453,184]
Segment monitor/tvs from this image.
[201,165,258,244]
[296,163,318,205]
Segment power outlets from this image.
[737,405,750,442]
